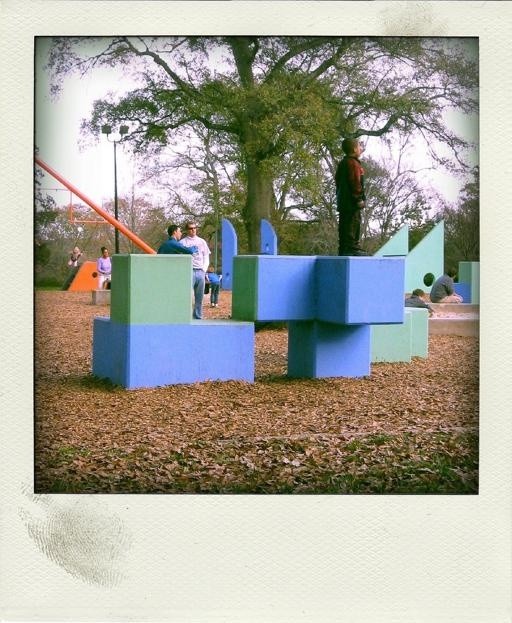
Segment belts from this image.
[193,268,203,270]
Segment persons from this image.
[406,289,435,319]
[205,266,222,307]
[97,246,111,290]
[430,268,463,303]
[335,138,367,256]
[67,246,83,268]
[157,225,198,254]
[178,223,211,319]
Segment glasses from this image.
[189,227,196,230]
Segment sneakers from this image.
[339,247,368,256]
[212,303,218,307]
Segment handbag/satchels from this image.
[204,283,209,294]
[106,281,111,289]
